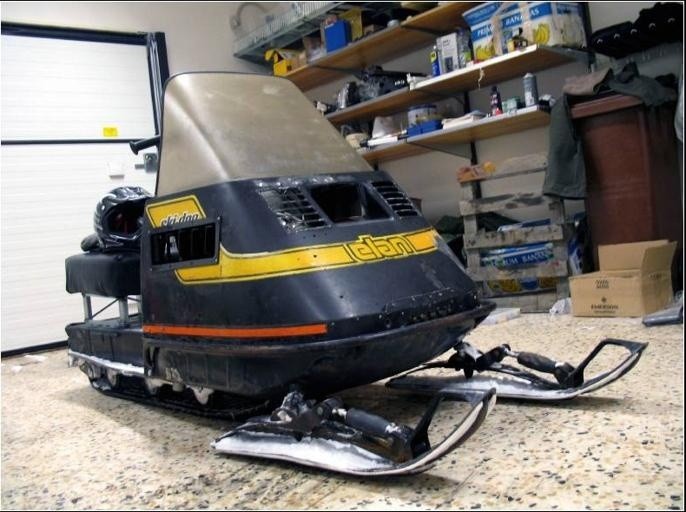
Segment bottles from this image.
[489,85,503,116]
[522,70,538,107]
[430,44,440,76]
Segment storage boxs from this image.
[481,216,583,297]
[463,2,588,63]
[568,238,679,317]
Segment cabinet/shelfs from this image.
[234,2,588,162]
